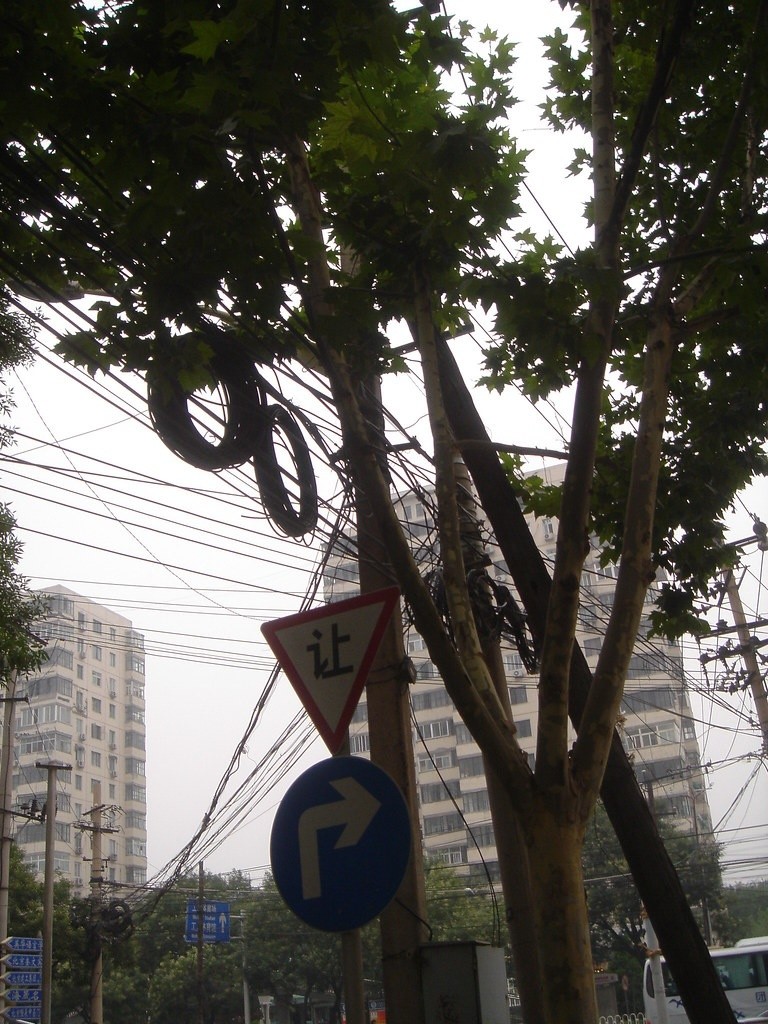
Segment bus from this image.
[644,936,768,1024]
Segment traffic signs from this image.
[0,936,44,1019]
